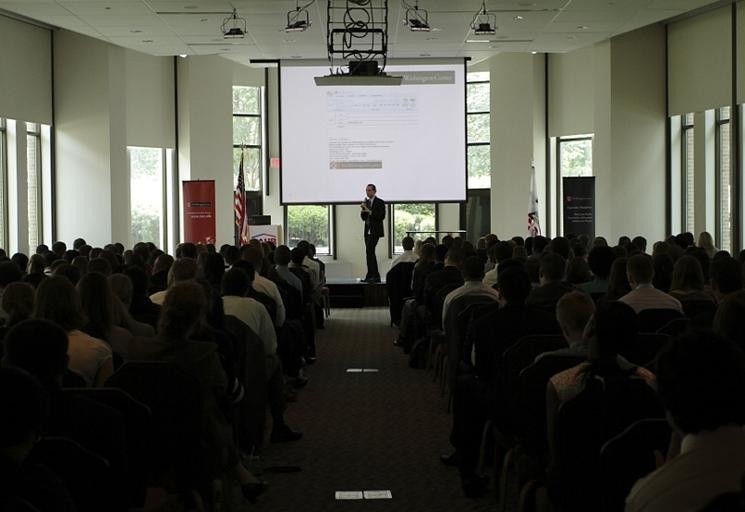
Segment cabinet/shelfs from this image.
[405,231,467,241]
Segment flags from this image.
[232,154,251,245]
[528,197,541,237]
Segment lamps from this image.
[399,0,431,34]
[219,7,247,39]
[279,0,312,32]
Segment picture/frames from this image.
[469,1,498,36]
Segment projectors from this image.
[314,61,403,85]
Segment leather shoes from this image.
[361,276,381,283]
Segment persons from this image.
[1,238,323,510]
[389,233,745,510]
[359,183,386,284]
